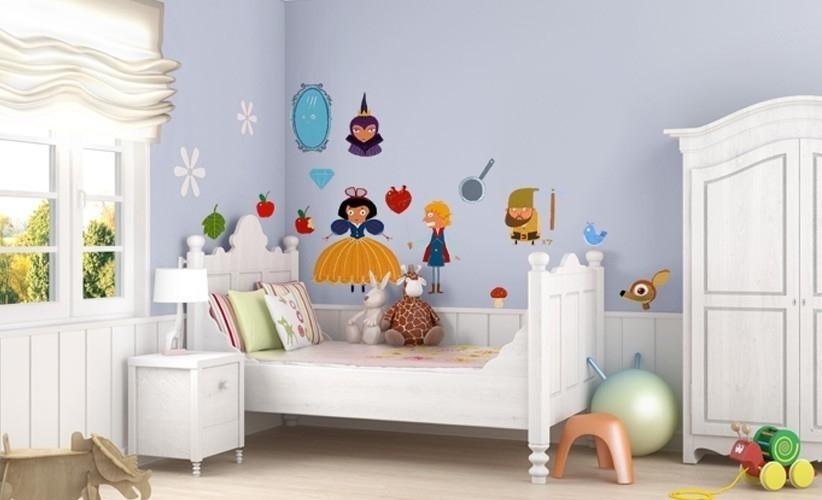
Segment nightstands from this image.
[129,351,244,477]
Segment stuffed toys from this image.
[341,269,393,343]
[379,264,449,351]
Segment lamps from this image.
[152,267,211,356]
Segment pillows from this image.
[209,279,324,353]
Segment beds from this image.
[186,216,607,486]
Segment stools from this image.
[554,414,635,486]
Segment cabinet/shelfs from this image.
[662,94,822,467]
